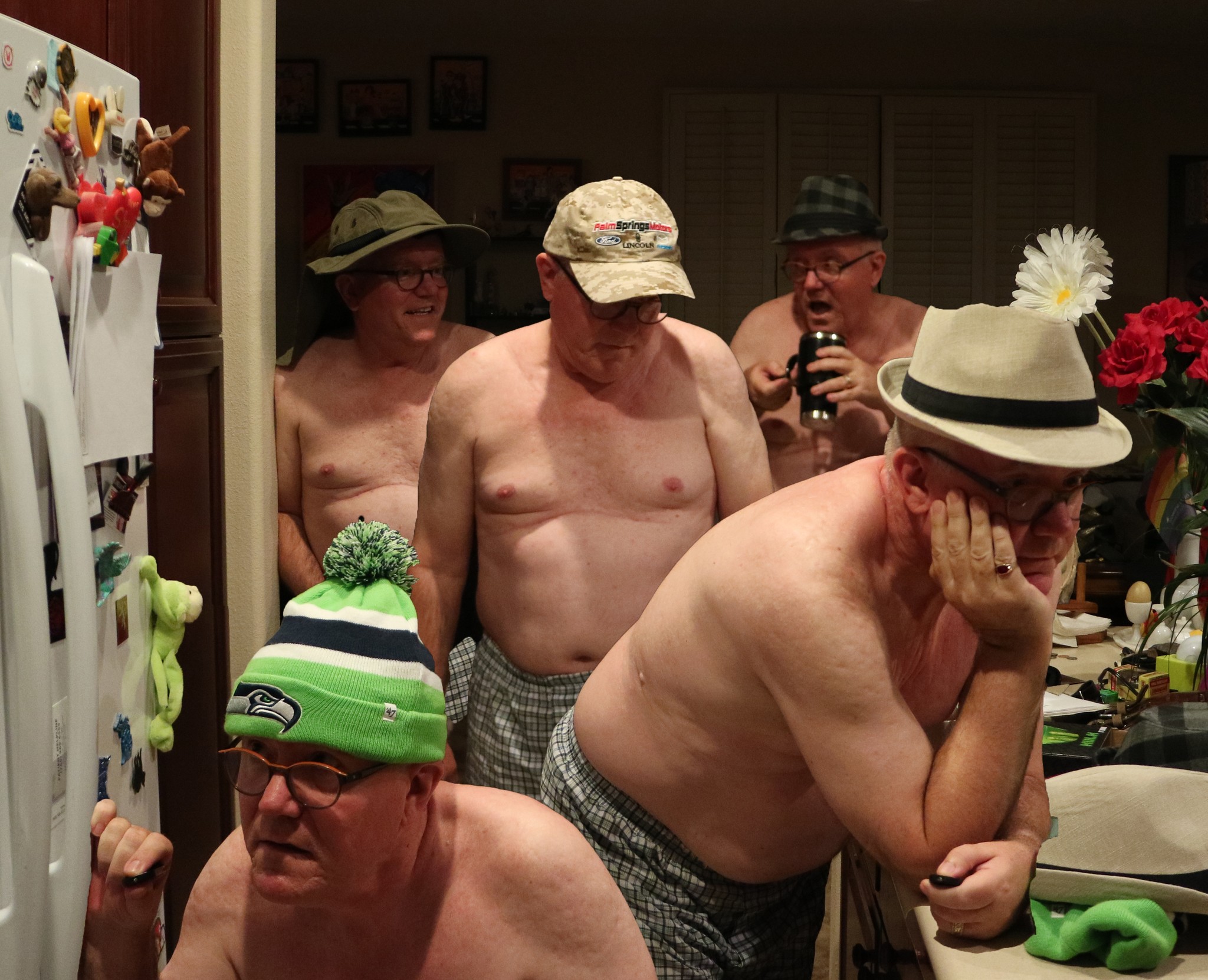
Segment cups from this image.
[785,330,848,431]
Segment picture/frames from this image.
[276,59,320,133]
[429,55,487,132]
[337,78,413,137]
[299,160,437,270]
[501,158,582,222]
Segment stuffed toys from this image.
[17,117,190,272]
[135,552,203,752]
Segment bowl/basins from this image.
[1176,635,1208,664]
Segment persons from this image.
[74,519,657,980]
[728,174,934,495]
[537,301,1134,980]
[275,188,497,785]
[412,178,770,799]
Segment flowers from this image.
[1009,225,1208,529]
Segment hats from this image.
[305,191,495,274]
[223,522,447,763]
[876,301,1134,469]
[541,177,696,303]
[765,173,889,244]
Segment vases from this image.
[1160,528,1204,630]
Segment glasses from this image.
[915,445,1097,524]
[555,257,675,324]
[217,738,394,809]
[354,267,452,288]
[779,251,882,284]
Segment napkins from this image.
[1052,610,1112,647]
[1044,691,1112,718]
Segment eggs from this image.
[1126,581,1151,603]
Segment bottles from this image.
[1170,616,1194,633]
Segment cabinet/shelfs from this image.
[465,238,550,332]
[658,85,1097,344]
[838,828,938,980]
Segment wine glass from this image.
[1146,603,1173,649]
[1124,599,1152,645]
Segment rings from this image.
[952,921,965,938]
[995,562,1019,577]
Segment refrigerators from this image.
[1,12,167,979]
